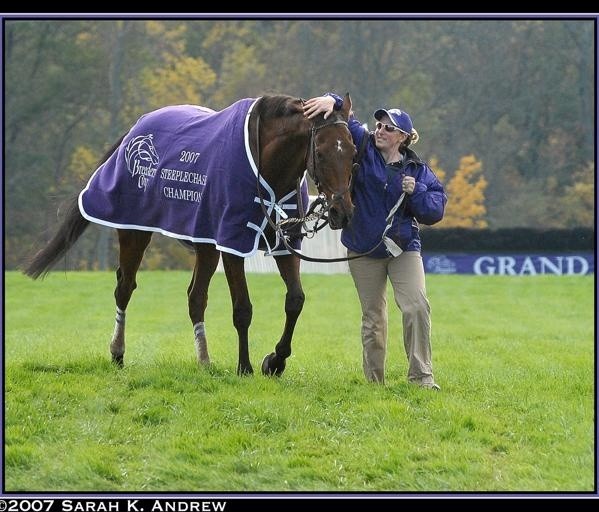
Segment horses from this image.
[19,92,357,377]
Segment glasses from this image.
[375,121,408,134]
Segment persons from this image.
[300,88,449,394]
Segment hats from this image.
[374,108,412,132]
